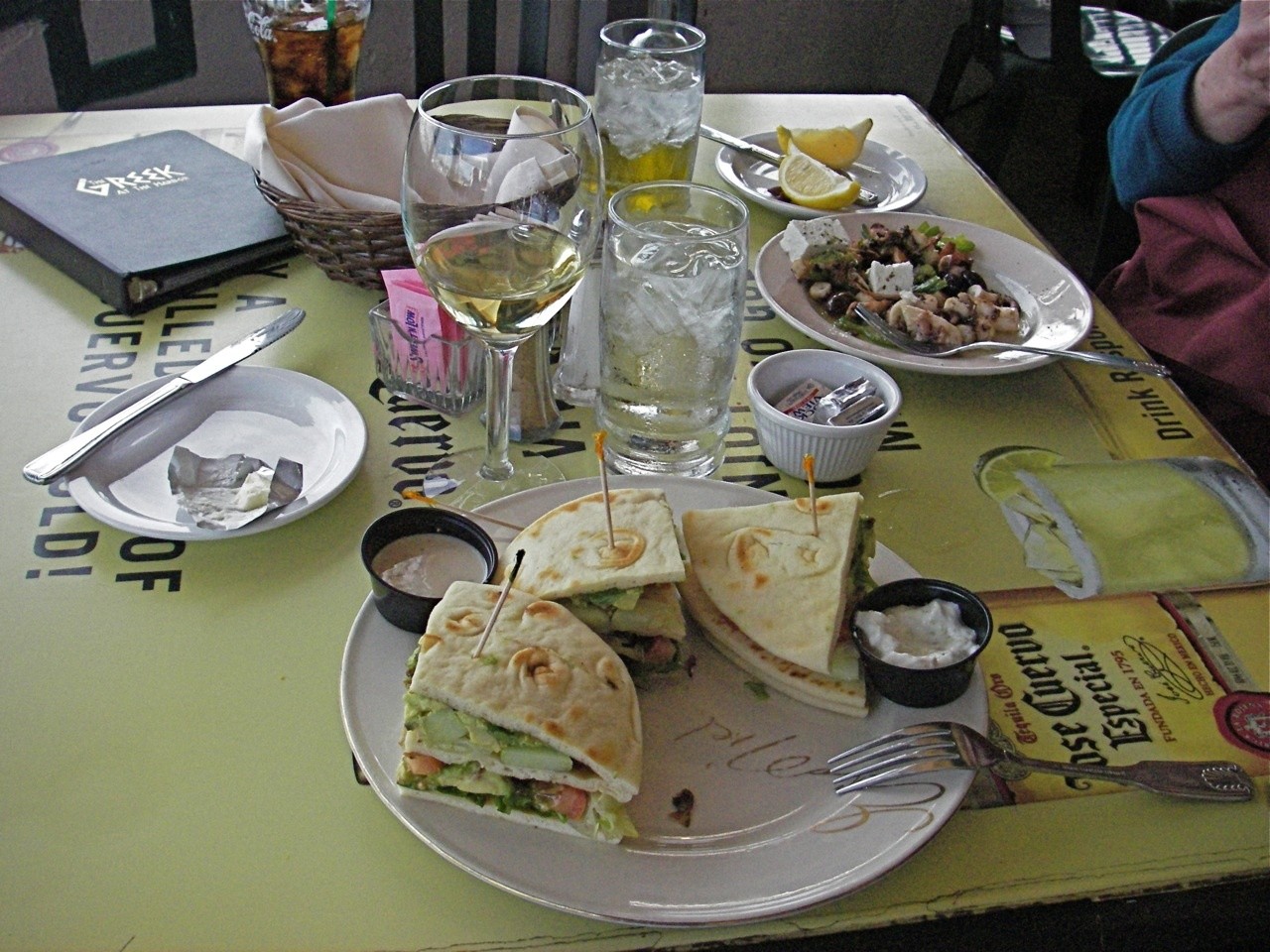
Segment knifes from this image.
[699,123,879,207]
[21,307,307,486]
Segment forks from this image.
[852,300,1172,379]
[826,720,1255,801]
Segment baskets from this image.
[255,113,584,290]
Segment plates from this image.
[755,211,1095,375]
[64,364,369,542]
[716,128,928,215]
[339,474,990,930]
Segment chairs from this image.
[412,0,703,106]
[1089,14,1222,289]
[927,0,1176,288]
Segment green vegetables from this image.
[801,221,974,352]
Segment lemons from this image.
[976,445,1063,503]
[776,117,875,168]
[780,139,860,209]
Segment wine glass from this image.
[399,73,607,514]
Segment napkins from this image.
[242,92,578,210]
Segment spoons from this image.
[568,200,614,261]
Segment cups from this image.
[747,349,903,483]
[240,0,372,110]
[594,17,707,221]
[593,180,749,479]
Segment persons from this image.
[1108,1,1270,497]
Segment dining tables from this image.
[0,95,1270,952]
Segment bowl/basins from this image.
[849,577,994,710]
[359,508,499,635]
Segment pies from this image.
[398,486,877,845]
